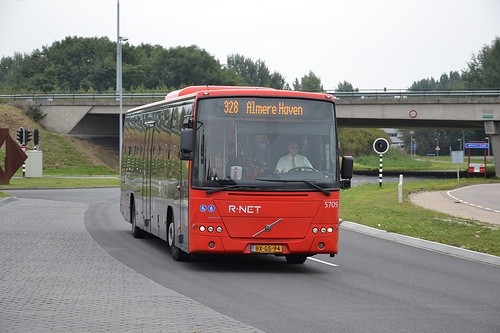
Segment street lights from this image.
[118,37,130,179]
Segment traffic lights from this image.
[17,128,23,144]
[26,129,33,144]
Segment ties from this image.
[292,157,295,168]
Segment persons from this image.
[274,140,321,175]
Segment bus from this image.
[120,85,355,262]
